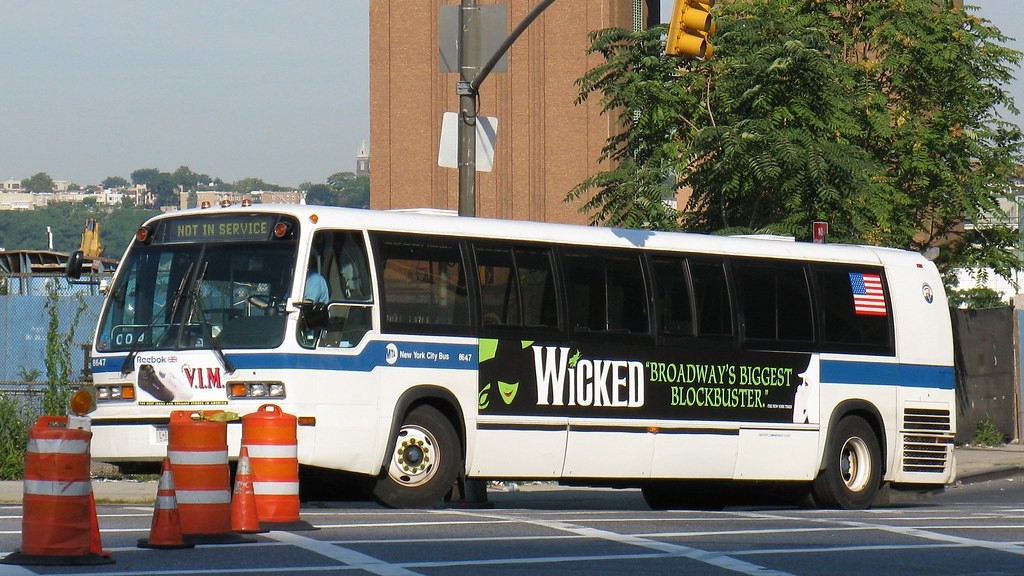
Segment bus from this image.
[65,198,959,508]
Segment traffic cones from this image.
[229,446,270,533]
[137,455,195,550]
[89,479,110,557]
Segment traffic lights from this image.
[666,0,717,60]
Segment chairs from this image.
[482,271,728,336]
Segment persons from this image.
[282,247,332,304]
